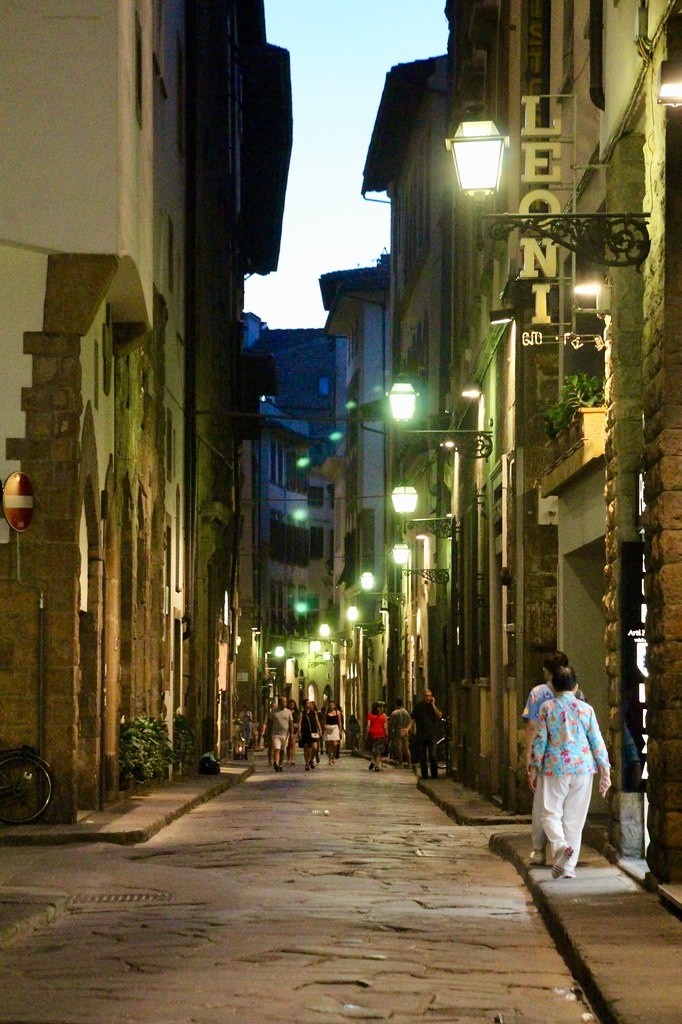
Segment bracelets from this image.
[600,767,610,772]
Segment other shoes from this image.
[328,759,336,765]
[273,763,284,772]
[529,851,546,865]
[551,846,579,879]
[305,762,315,770]
[369,762,380,772]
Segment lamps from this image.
[446,99,656,275]
[659,60,682,107]
[391,481,462,540]
[385,373,493,459]
[311,544,449,660]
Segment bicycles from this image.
[436,718,451,768]
[350,729,358,752]
[0,746,54,826]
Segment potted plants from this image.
[543,371,611,459]
[117,716,174,789]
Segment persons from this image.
[234,704,259,746]
[261,697,361,772]
[365,702,388,772]
[399,689,443,779]
[521,649,612,879]
[391,698,412,769]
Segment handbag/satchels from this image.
[311,733,320,738]
